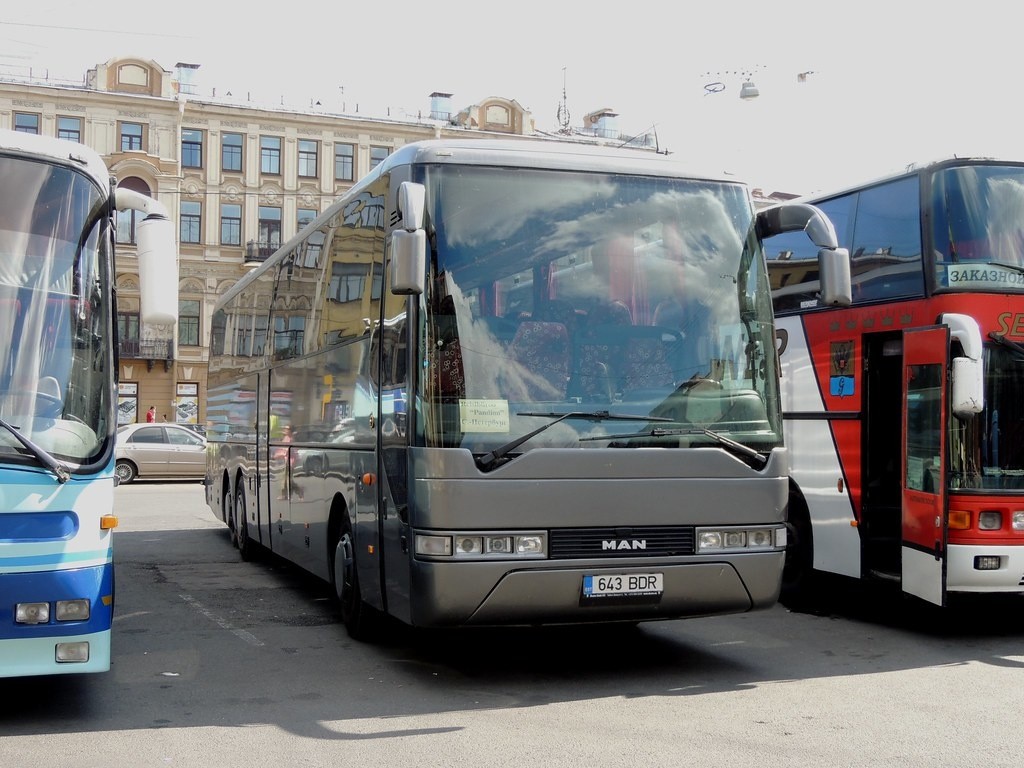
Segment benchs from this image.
[494,290,697,406]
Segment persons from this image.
[161,415,167,423]
[146,406,156,422]
[266,409,306,499]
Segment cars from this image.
[115,423,208,485]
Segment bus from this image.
[202,136,855,632]
[0,124,182,693]
[748,156,1024,622]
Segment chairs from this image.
[0,298,93,350]
